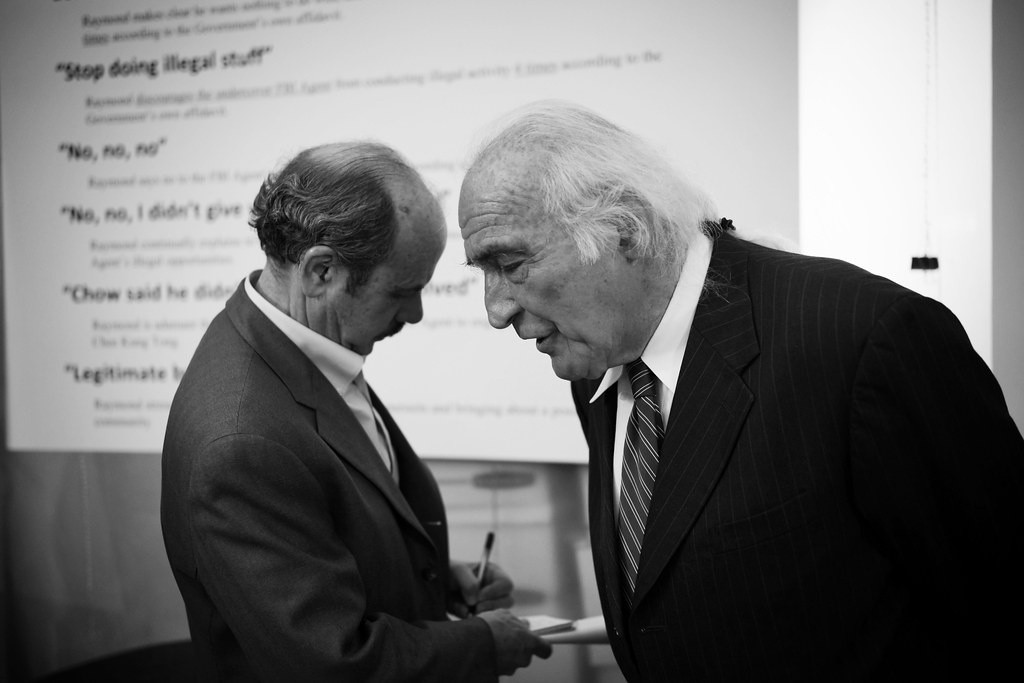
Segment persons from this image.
[459,102,1024,683]
[159,142,553,683]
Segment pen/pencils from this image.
[467,532,495,619]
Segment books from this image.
[517,613,573,635]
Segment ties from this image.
[616,356,664,613]
